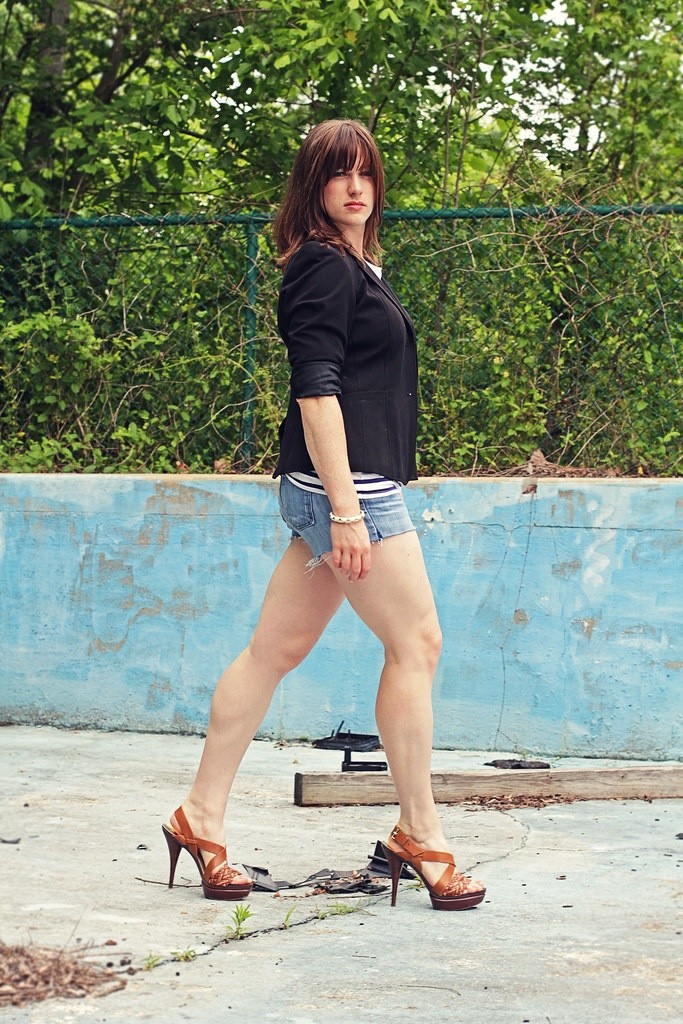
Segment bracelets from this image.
[328,510,366,525]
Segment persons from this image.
[158,118,489,911]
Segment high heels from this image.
[381,824,486,911]
[162,806,252,901]
